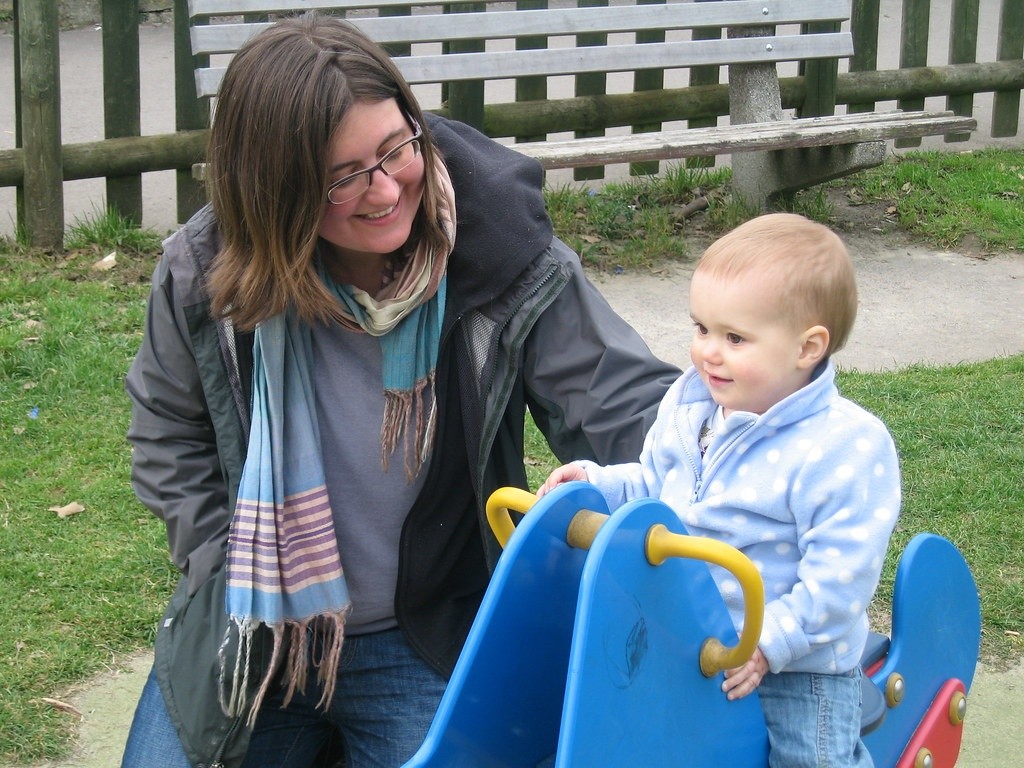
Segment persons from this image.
[530,209,908,767]
[110,12,683,767]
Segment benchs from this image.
[188,0,978,216]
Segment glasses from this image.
[322,107,423,205]
